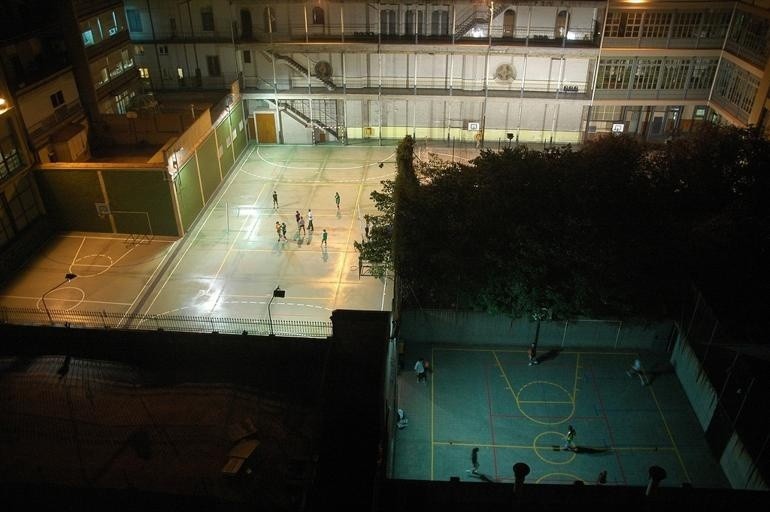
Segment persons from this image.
[528,341,540,367]
[470,446,479,476]
[564,422,577,452]
[334,191,341,211]
[413,356,427,384]
[275,209,315,243]
[273,191,279,209]
[321,229,328,246]
[631,357,653,386]
